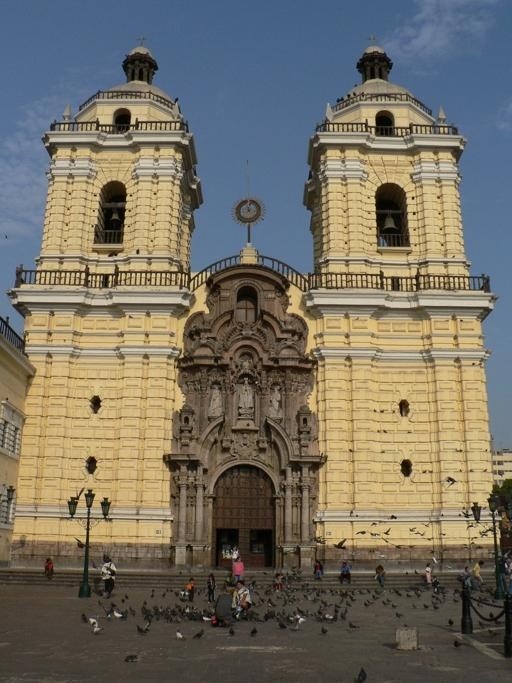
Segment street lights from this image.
[0,484,16,502]
[469,491,512,600]
[66,486,112,597]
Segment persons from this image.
[100,556,117,597]
[431,575,439,592]
[185,543,284,627]
[313,559,323,578]
[425,562,431,583]
[472,559,485,591]
[374,564,387,586]
[42,556,57,580]
[339,561,351,583]
[462,565,471,590]
[504,550,512,594]
[498,554,508,595]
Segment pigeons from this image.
[125,654,139,663]
[73,536,90,549]
[81,585,505,640]
[329,521,495,558]
